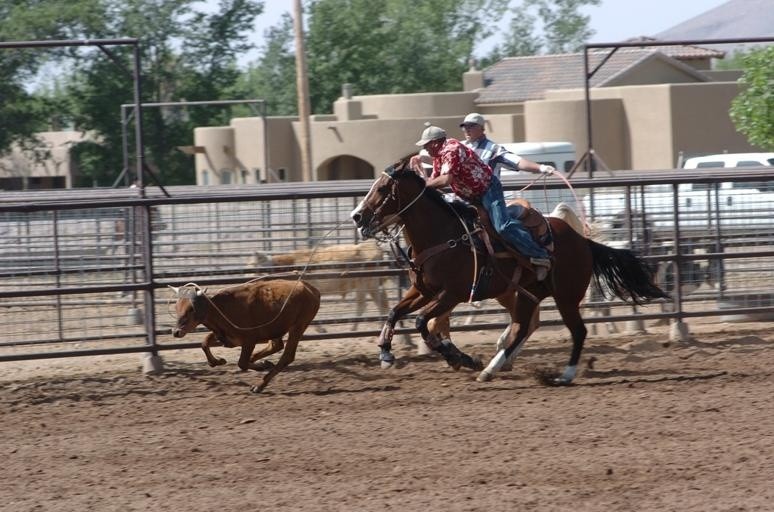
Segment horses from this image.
[348,159,670,387]
[105,206,169,255]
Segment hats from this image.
[459,113,484,127]
[416,126,446,147]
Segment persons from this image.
[416,113,556,185]
[130,178,140,188]
[416,126,549,282]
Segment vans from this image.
[495,139,577,215]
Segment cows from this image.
[241,240,389,334]
[166,276,321,394]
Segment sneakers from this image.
[537,266,547,281]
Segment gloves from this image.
[540,164,555,176]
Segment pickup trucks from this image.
[580,150,774,248]
